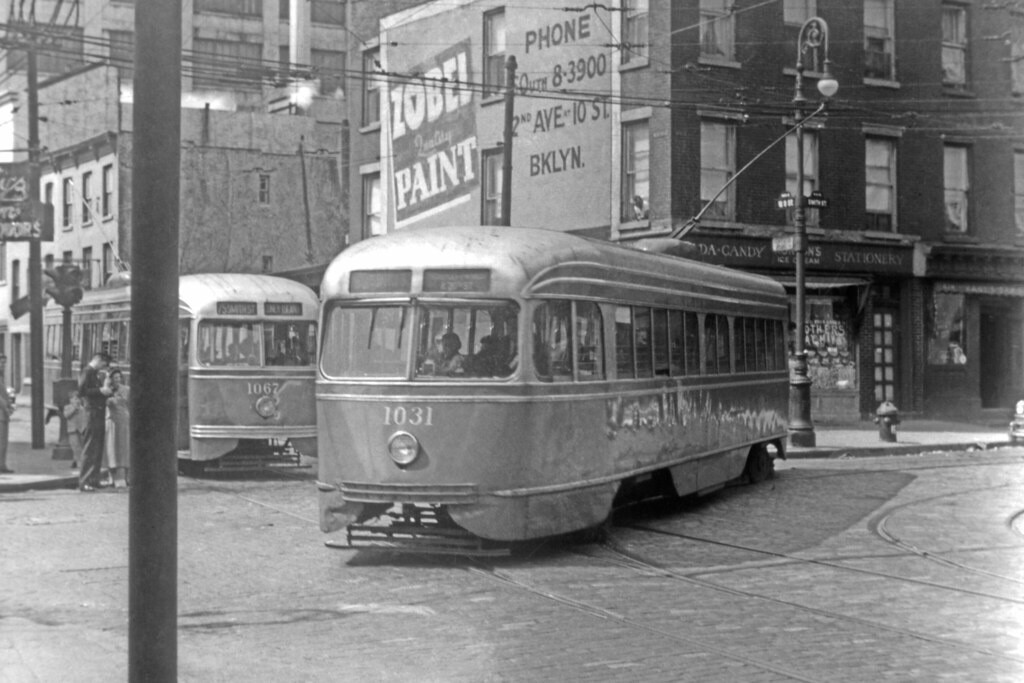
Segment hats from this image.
[93,352,113,362]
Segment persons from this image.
[475,335,501,361]
[75,352,112,493]
[438,332,465,374]
[63,391,84,468]
[240,338,260,366]
[271,337,300,366]
[104,370,130,488]
[224,344,240,365]
[0,353,15,474]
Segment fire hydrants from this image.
[873,401,899,443]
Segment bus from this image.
[316,224,791,553]
[39,272,322,476]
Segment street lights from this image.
[781,16,837,449]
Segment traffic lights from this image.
[44,265,65,306]
[66,267,82,305]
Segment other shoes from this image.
[104,484,115,488]
[0,469,15,474]
[89,484,105,488]
[79,485,94,492]
